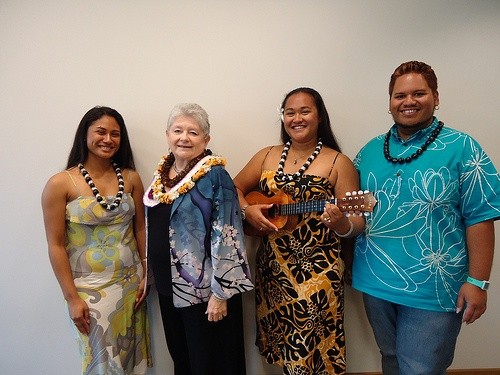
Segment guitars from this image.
[243,189,378,235]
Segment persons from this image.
[42,105,154,375]
[143,101,256,375]
[232,87,369,375]
[351,61,500,375]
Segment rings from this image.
[74,323,77,325]
[324,217,332,223]
[259,228,263,230]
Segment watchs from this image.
[241,206,248,220]
[467,276,491,291]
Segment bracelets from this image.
[334,220,354,238]
[140,259,146,261]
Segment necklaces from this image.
[77,159,125,210]
[145,149,227,207]
[383,121,444,164]
[278,137,322,182]
